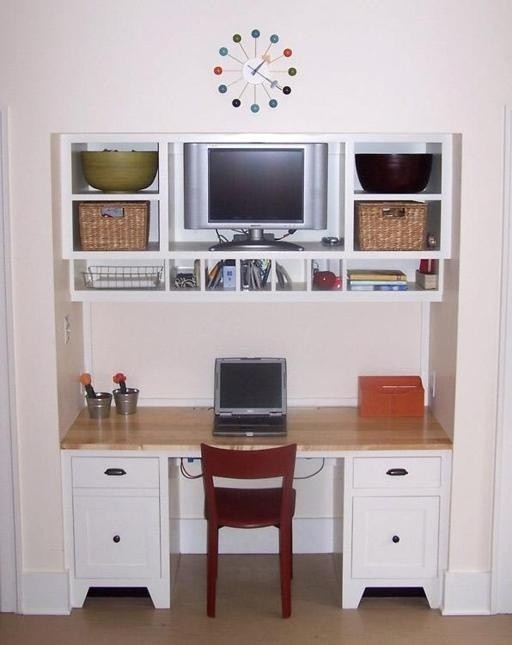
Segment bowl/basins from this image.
[80,151,159,195]
[355,153,432,193]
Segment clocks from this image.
[214,28,296,112]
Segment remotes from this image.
[322,236,338,245]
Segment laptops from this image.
[213,357,287,438]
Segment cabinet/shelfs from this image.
[59,134,453,302]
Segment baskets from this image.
[82,265,165,290]
[357,203,432,252]
[77,202,150,251]
[357,375,425,420]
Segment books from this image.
[347,270,409,290]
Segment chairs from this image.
[200,442,296,618]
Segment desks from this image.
[60,406,453,610]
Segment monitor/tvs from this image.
[184,142,328,250]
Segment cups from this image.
[112,388,139,414]
[86,392,113,419]
